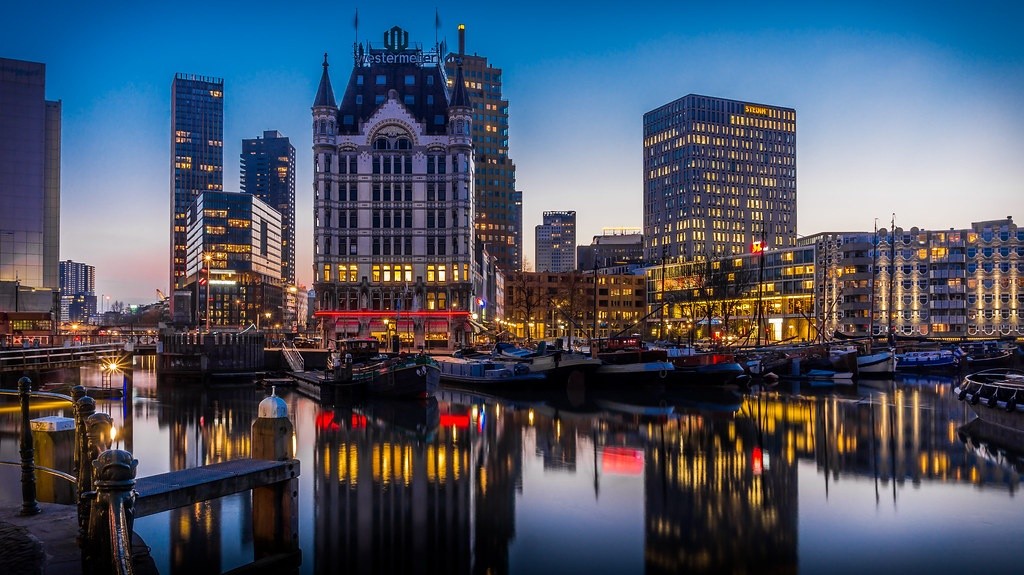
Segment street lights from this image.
[206,254,211,331]
[265,312,272,349]
[383,318,389,353]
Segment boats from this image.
[667,348,744,386]
[598,350,676,407]
[857,347,899,372]
[326,336,441,400]
[492,342,602,411]
[426,340,546,402]
